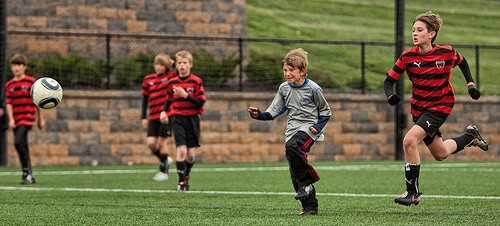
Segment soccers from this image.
[30,76,64,110]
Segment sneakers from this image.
[465,125,489,151]
[394,189,422,206]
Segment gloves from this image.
[388,94,400,106]
[468,86,481,100]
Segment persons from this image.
[141,51,206,192]
[383,11,489,206]
[248,48,331,216]
[5,55,45,184]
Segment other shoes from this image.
[167,156,172,165]
[153,172,168,181]
[294,184,314,200]
[298,207,317,216]
[20,168,35,184]
[177,176,190,191]
